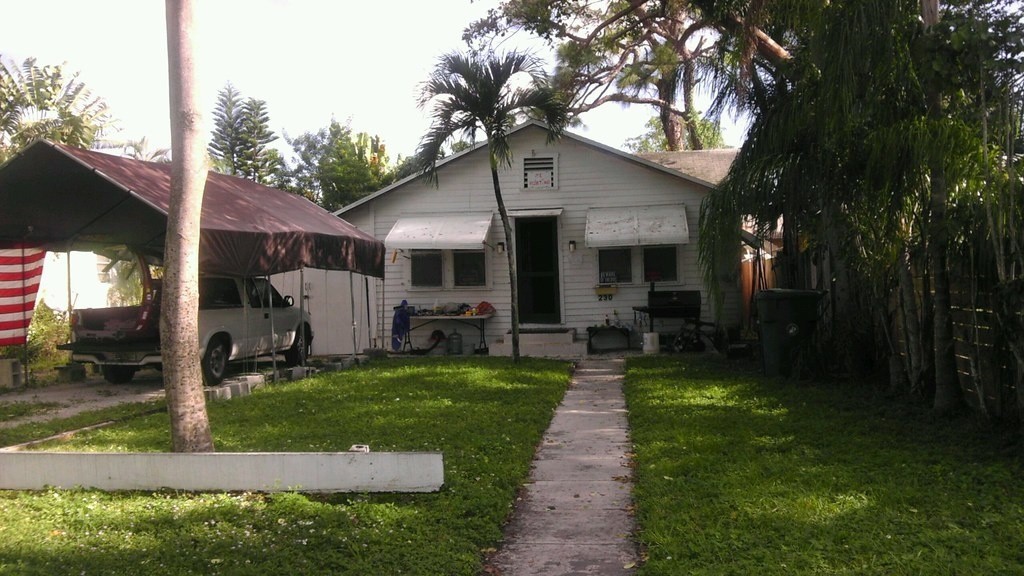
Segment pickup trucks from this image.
[56,265,316,387]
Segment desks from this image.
[586,327,632,352]
[394,306,493,354]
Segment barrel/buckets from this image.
[448,328,463,354]
[643,332,659,354]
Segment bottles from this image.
[433,298,442,314]
[604,314,609,326]
